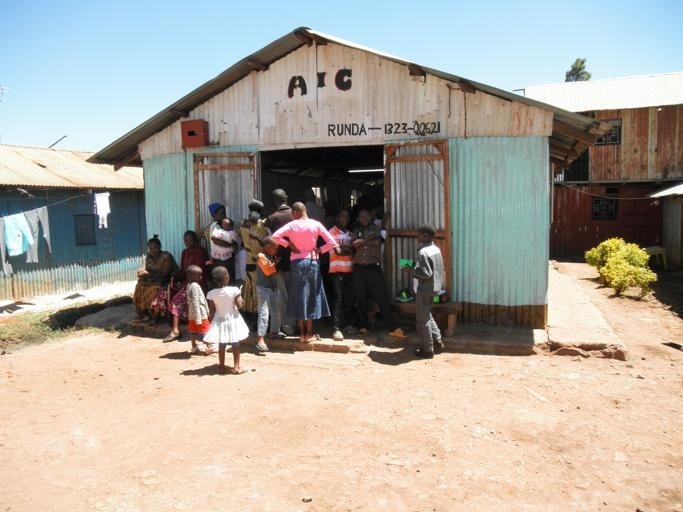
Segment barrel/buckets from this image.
[390,326,407,339]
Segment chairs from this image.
[131,315,219,353]
[435,342,446,352]
[415,349,435,359]
[255,327,370,352]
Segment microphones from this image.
[642,246,667,269]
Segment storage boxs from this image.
[388,299,462,339]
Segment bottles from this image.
[209,203,223,214]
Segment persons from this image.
[183,263,215,356]
[160,229,207,344]
[127,235,179,325]
[398,223,445,359]
[201,180,410,354]
[205,266,249,375]
